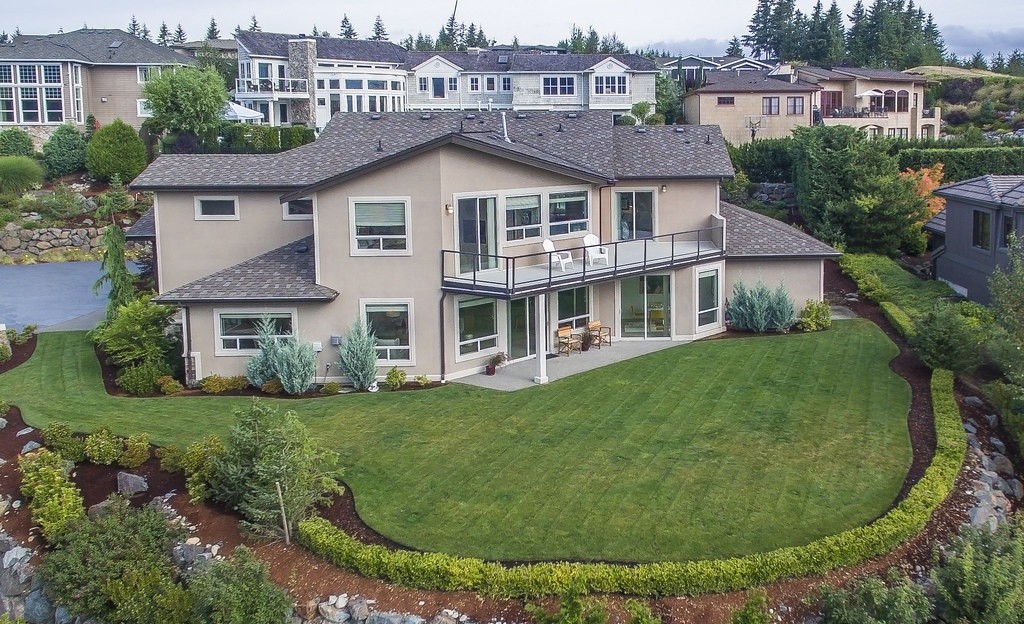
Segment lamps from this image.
[445,203,453,213]
[661,184,672,193]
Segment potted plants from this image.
[486,350,511,376]
[580,330,593,351]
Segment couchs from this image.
[374,336,408,359]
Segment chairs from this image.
[588,319,611,349]
[631,305,643,321]
[582,233,609,267]
[246,81,257,92]
[831,105,870,118]
[543,239,574,272]
[557,325,581,358]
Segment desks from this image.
[648,307,664,325]
